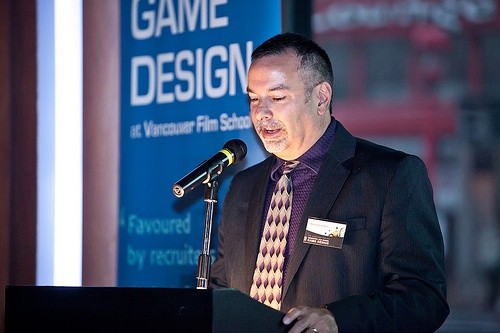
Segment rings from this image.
[311,328,319,333]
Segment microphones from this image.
[172,139,248,199]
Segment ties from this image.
[248,161,300,311]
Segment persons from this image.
[208,33,450,333]
